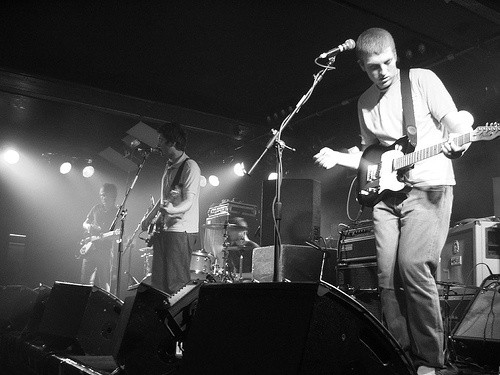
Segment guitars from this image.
[355,122,500,206]
[146,188,180,247]
[74,229,121,258]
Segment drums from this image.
[145,254,154,275]
[188,251,214,276]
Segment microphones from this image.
[318,39,356,59]
[137,146,160,153]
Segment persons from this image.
[226,216,260,273]
[151,129,201,296]
[312,29,473,375]
[80,183,120,292]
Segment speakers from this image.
[0,286,41,333]
[110,283,197,375]
[185,281,417,375]
[450,275,500,362]
[43,281,123,357]
[262,179,321,245]
[335,262,408,331]
